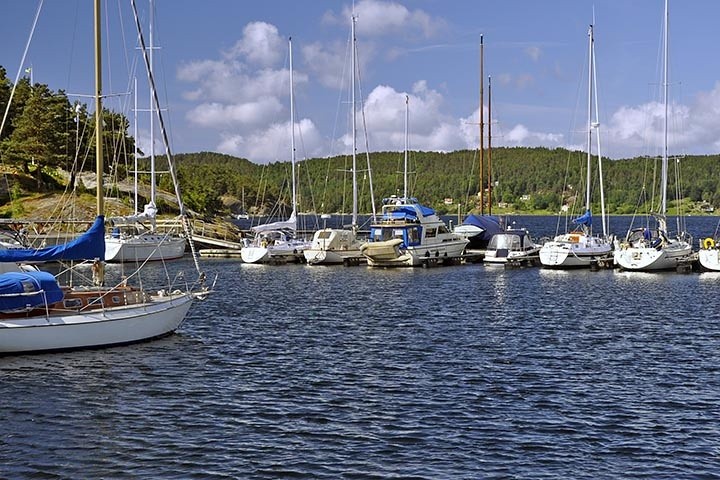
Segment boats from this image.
[484,226,543,267]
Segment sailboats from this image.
[539,3,616,267]
[0,0,219,352]
[239,8,508,270]
[74,0,187,262]
[609,0,696,274]
[697,213,720,272]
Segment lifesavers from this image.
[704,238,714,249]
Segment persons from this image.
[641,228,652,242]
